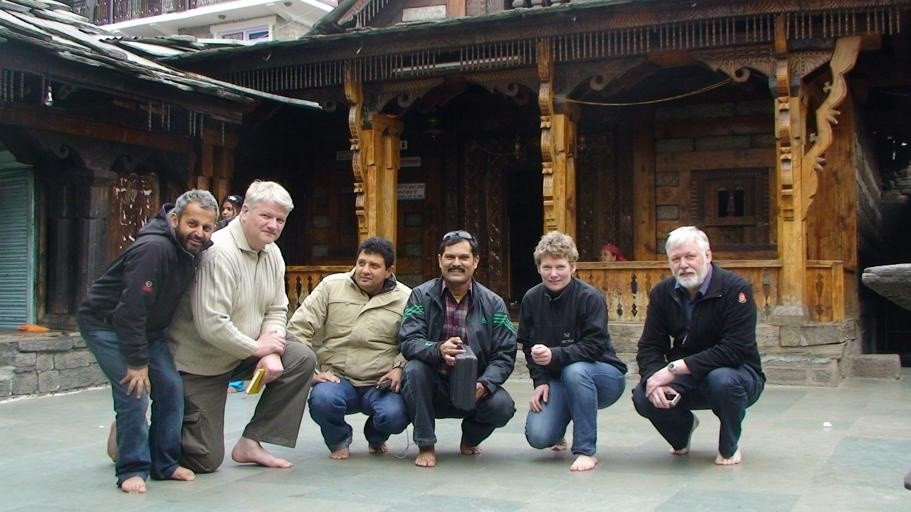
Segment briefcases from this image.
[450,344,478,410]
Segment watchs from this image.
[667,361,680,378]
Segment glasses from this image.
[441,229,475,242]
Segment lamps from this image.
[44,85,54,107]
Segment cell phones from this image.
[664,394,681,406]
[377,379,391,390]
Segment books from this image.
[246,368,265,394]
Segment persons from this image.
[108,179,318,468]
[598,243,626,262]
[76,187,221,495]
[516,229,627,472]
[286,236,413,460]
[218,194,247,395]
[630,225,766,466]
[396,230,517,469]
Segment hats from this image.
[224,194,243,205]
